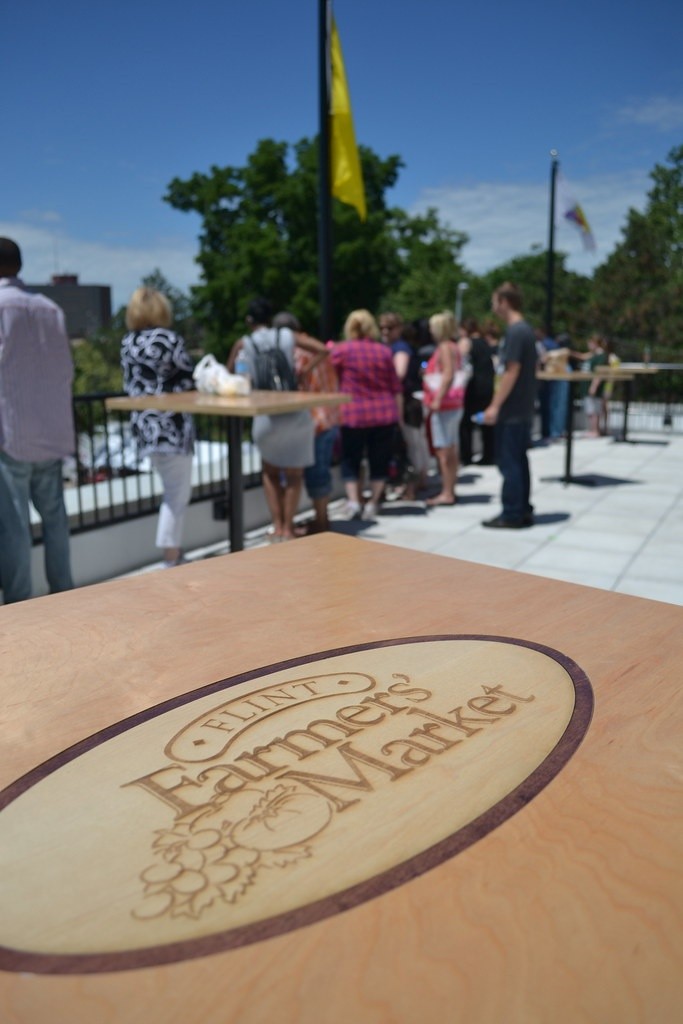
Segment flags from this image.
[555,170,596,254]
[329,3,367,221]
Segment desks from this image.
[596,364,657,443]
[535,372,633,487]
[651,362,683,427]
[106,391,351,553]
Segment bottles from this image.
[234,349,251,381]
[471,412,497,425]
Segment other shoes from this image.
[328,500,377,521]
[262,533,298,543]
[483,513,532,528]
[293,520,328,537]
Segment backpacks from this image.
[249,326,298,418]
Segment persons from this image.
[121,287,197,566]
[273,313,340,530]
[228,301,329,543]
[481,281,539,529]
[453,311,621,464]
[376,310,426,503]
[327,309,396,521]
[418,314,465,505]
[0,236,74,604]
